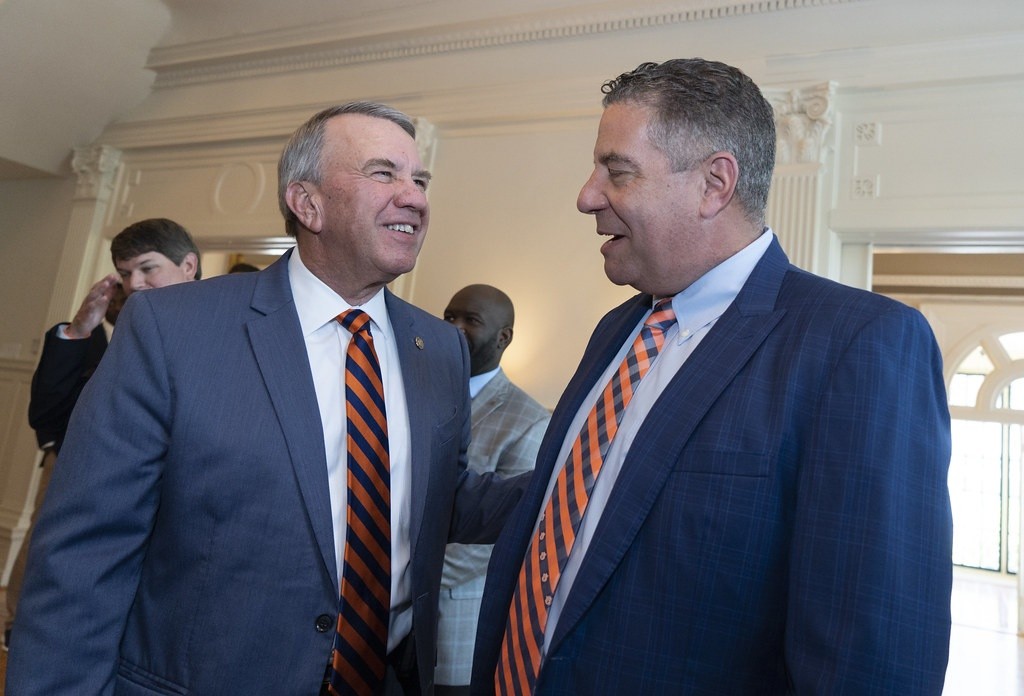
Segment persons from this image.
[468,57,952,696]
[432,283,554,696]
[0,102,538,696]
[0,282,129,652]
[28,218,202,459]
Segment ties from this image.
[328,310,391,696]
[489,297,676,696]
